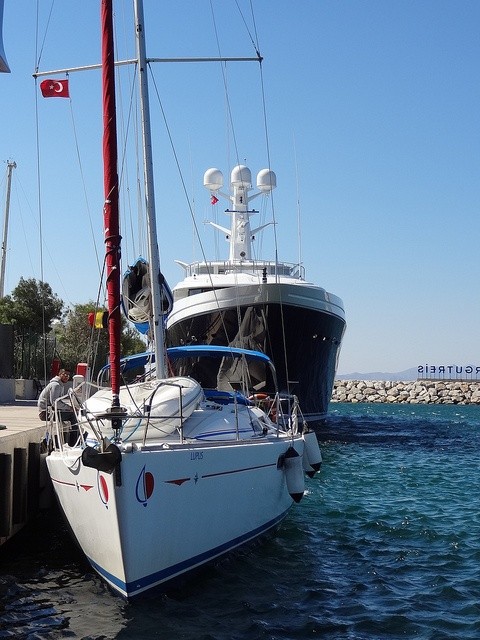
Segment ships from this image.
[163,164,347,429]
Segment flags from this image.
[39,78,70,99]
[211,194,219,204]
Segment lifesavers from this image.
[247,394,276,424]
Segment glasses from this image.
[60,372,70,377]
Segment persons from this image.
[37,368,83,447]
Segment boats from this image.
[33,2,323,601]
